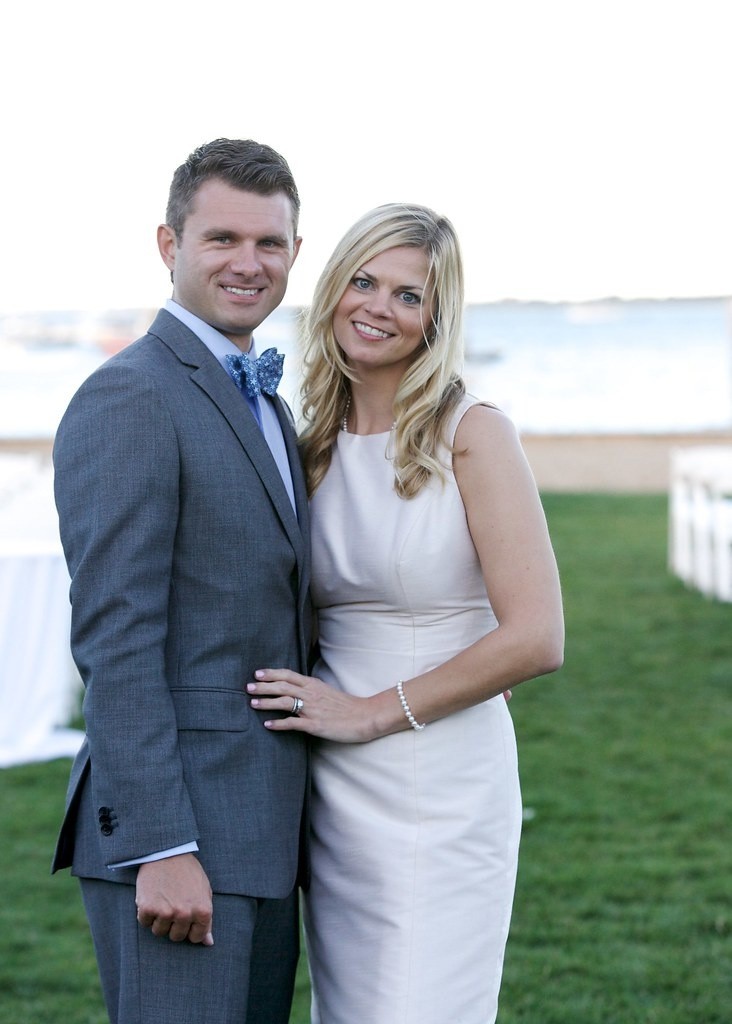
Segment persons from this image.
[43,137,511,1023]
[241,203,574,1023]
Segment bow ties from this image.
[226,345,285,397]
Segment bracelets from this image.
[398,680,425,733]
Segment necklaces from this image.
[343,394,399,438]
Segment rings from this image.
[293,695,305,712]
